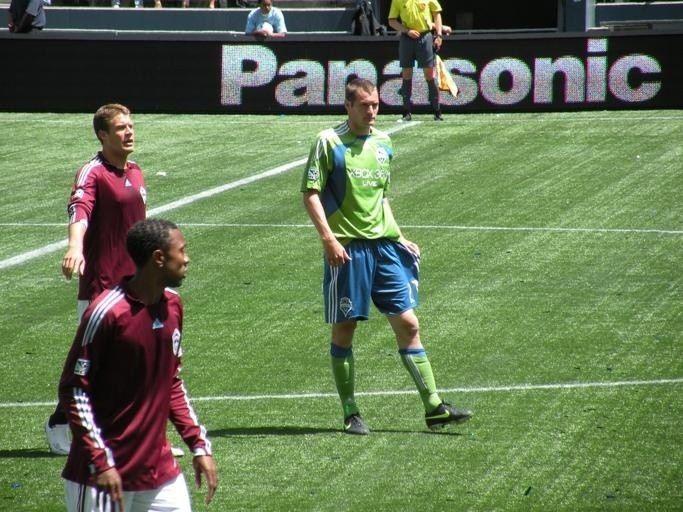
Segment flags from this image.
[433,53,459,97]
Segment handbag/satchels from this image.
[351,0,384,36]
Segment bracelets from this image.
[435,34,442,38]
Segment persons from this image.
[243,1,288,37]
[9,0,50,34]
[46,104,146,456]
[88,1,247,7]
[61,219,218,511]
[301,79,472,436]
[432,22,453,35]
[388,0,443,122]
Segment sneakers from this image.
[425,399,474,428]
[344,412,370,434]
[403,110,411,120]
[46,414,70,455]
[433,109,443,121]
